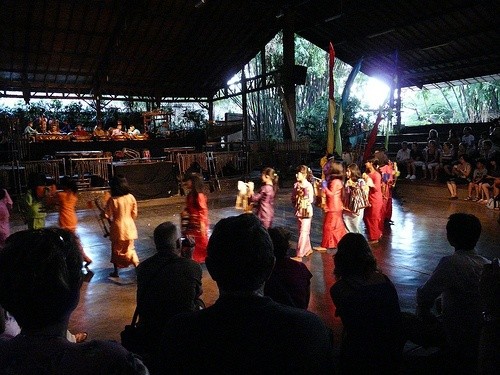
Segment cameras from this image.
[177,238,188,248]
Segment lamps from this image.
[194,0,205,8]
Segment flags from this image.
[328,42,393,164]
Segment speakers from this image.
[290,64,307,85]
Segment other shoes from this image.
[449,196,458,199]
[477,198,495,209]
[464,196,472,200]
[472,197,479,201]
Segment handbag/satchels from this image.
[341,186,371,213]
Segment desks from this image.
[108,158,179,199]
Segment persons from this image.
[24,120,70,142]
[396,117,500,180]
[0,171,92,268]
[93,119,140,136]
[136,221,203,320]
[69,123,92,137]
[330,233,408,375]
[120,212,335,375]
[402,213,492,347]
[103,173,138,277]
[447,155,500,210]
[0,226,149,375]
[290,151,400,262]
[264,228,313,310]
[180,169,210,263]
[250,168,279,230]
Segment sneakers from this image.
[406,174,416,179]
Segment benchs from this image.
[360,125,448,153]
